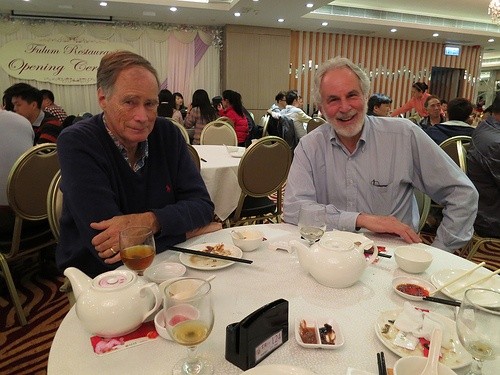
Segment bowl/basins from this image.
[154,304,200,341]
[393,356,457,375]
[230,229,264,252]
[394,246,432,273]
[159,277,211,307]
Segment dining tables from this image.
[189,145,248,221]
[47,223,500,375]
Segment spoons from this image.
[171,274,215,300]
[420,329,442,375]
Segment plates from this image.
[179,242,243,271]
[143,263,186,283]
[392,276,436,301]
[316,231,373,251]
[430,266,500,308]
[375,306,472,369]
[295,317,344,349]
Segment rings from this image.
[110,247,117,254]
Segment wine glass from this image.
[165,278,215,375]
[457,288,500,375]
[119,226,155,276]
[298,203,326,245]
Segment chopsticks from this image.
[376,352,387,375]
[201,158,207,162]
[301,235,392,259]
[162,246,253,264]
[422,296,500,316]
[430,262,500,296]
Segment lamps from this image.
[10,9,115,24]
[487,0,500,25]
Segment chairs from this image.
[228,136,292,225]
[418,136,472,233]
[200,121,237,146]
[456,140,500,261]
[1,143,61,326]
[263,115,326,149]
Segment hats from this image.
[210,96,222,105]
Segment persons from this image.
[390,82,446,118]
[158,89,187,127]
[466,92,500,218]
[267,89,312,146]
[282,58,480,254]
[184,89,219,145]
[58,51,222,282]
[465,97,493,126]
[0,103,35,240]
[212,90,255,148]
[366,93,392,117]
[424,98,477,145]
[417,96,448,129]
[3,83,68,144]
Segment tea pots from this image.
[63,267,162,338]
[289,235,378,288]
